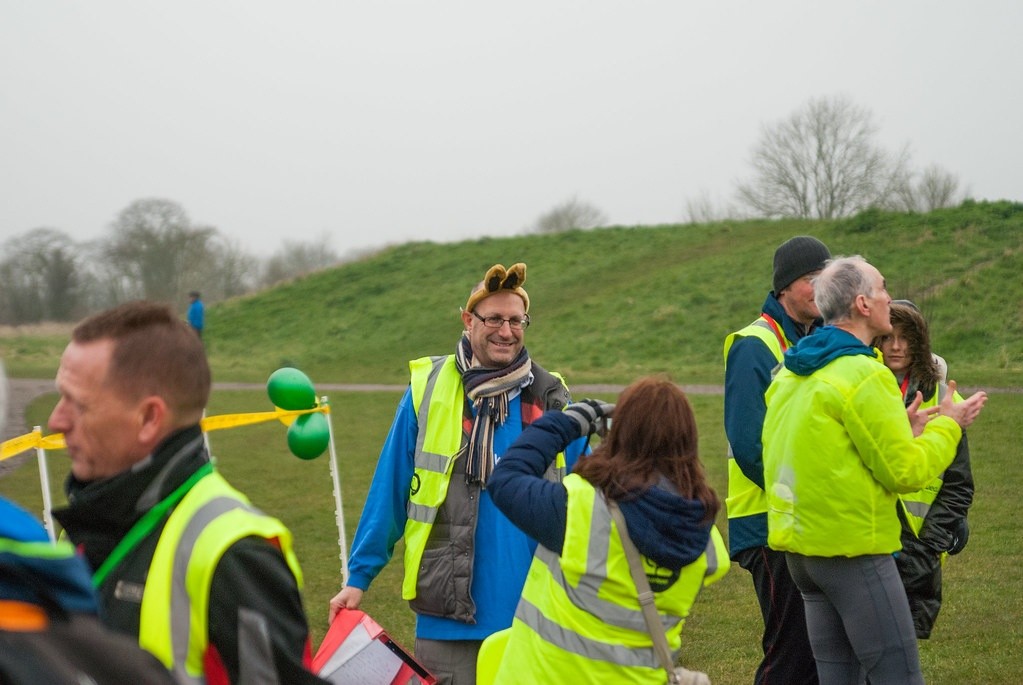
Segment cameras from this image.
[596,417,613,431]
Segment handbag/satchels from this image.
[666,663,713,685]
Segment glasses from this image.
[471,306,530,330]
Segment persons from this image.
[48,304,309,685]
[327,279,591,685]
[186,291,204,333]
[476,373,731,685]
[761,258,988,685]
[723,236,831,685]
[871,300,975,640]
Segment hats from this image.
[772,237,833,296]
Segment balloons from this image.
[267,368,315,410]
[287,411,330,460]
[275,395,321,426]
